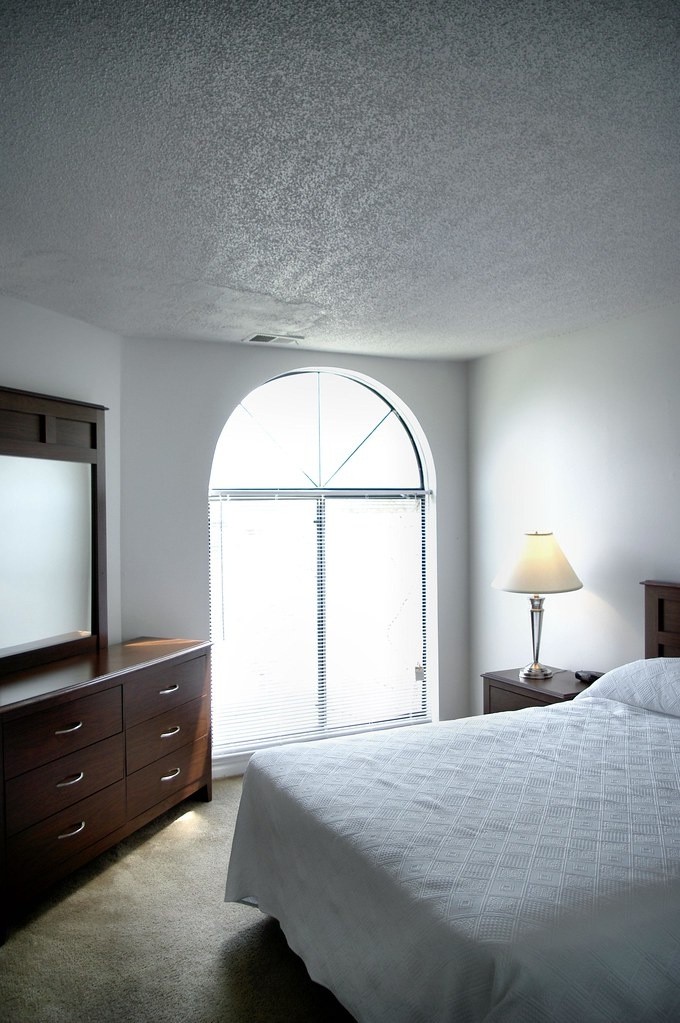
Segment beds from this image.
[230,580,680,1023]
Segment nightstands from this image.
[479,665,591,714]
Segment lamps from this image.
[490,532,584,681]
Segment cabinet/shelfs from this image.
[0,636,216,947]
